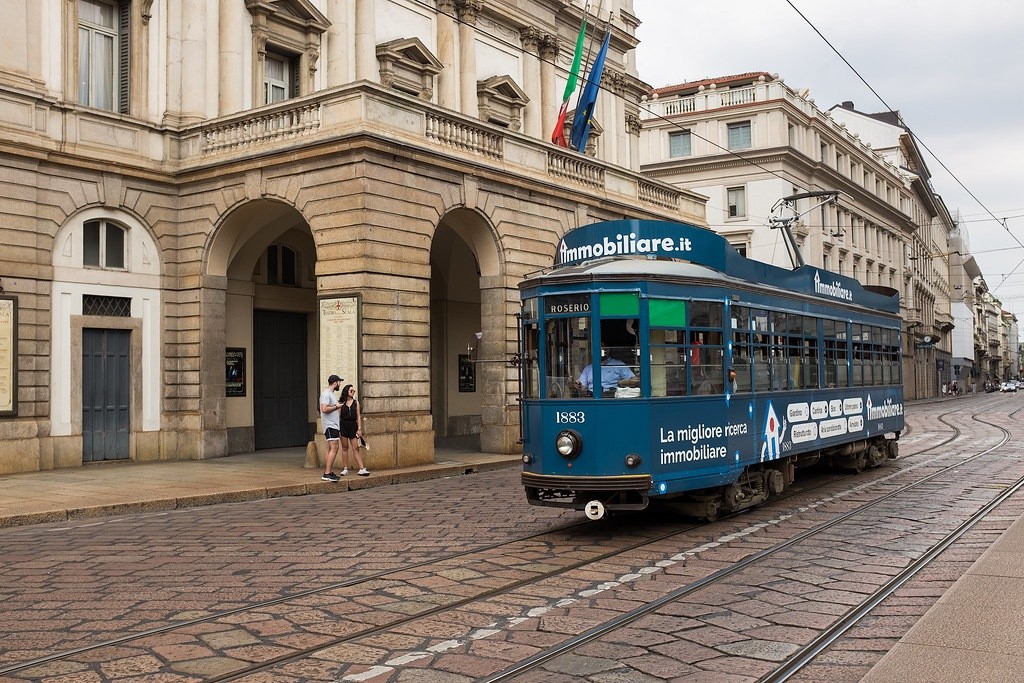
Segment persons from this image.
[338,385,370,476]
[942,382,959,397]
[666,361,711,394]
[320,375,344,482]
[575,342,641,397]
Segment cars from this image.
[1000,380,1024,393]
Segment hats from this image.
[328,375,344,385]
[357,436,370,451]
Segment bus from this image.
[514,189,904,525]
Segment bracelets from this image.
[358,427,362,430]
[334,405,337,410]
[628,378,631,385]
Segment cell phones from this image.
[355,433,359,438]
[340,402,345,405]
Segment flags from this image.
[572,32,610,152]
[551,21,587,148]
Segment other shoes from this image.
[357,466,370,476]
[321,471,341,482]
[339,470,349,476]
